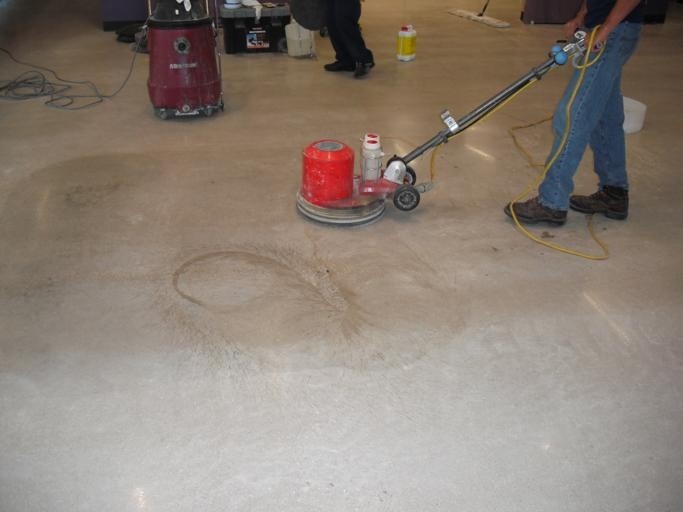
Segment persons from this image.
[324,0,375,78]
[504,0,668,226]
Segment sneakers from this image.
[569,185,628,219]
[324,58,356,71]
[503,197,568,225]
[354,60,375,78]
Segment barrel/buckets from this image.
[398,26,417,61]
[285,18,314,57]
